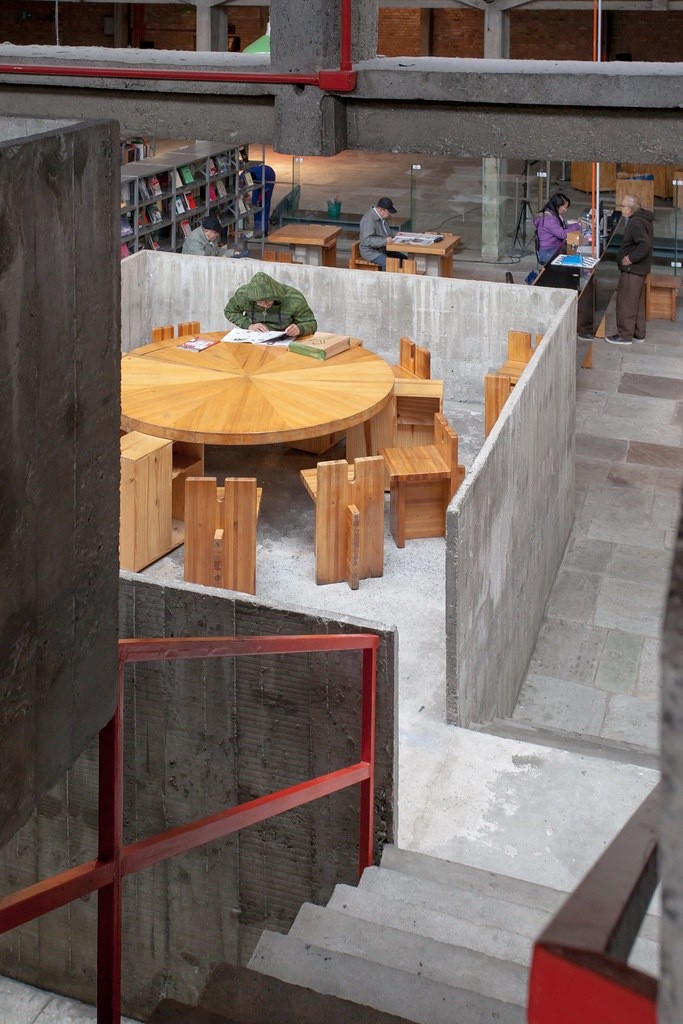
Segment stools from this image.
[121,321,546,595]
[645,271,682,322]
[347,242,380,272]
[385,257,425,276]
[264,251,303,264]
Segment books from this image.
[551,254,600,268]
[121,141,254,259]
[231,250,249,258]
[176,329,350,361]
[392,235,445,247]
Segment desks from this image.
[268,224,343,265]
[386,233,461,277]
[120,328,395,446]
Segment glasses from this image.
[256,300,270,305]
[560,205,567,212]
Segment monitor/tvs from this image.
[599,205,609,237]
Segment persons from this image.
[239,165,276,239]
[533,193,581,262]
[182,216,242,258]
[224,272,317,336]
[605,194,655,345]
[359,197,407,272]
[424,161,463,255]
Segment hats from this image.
[377,198,397,213]
[202,216,223,232]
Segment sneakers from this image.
[605,334,632,344]
[632,335,645,342]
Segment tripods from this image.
[513,159,534,251]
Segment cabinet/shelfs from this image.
[120,140,264,261]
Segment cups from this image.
[328,201,340,216]
